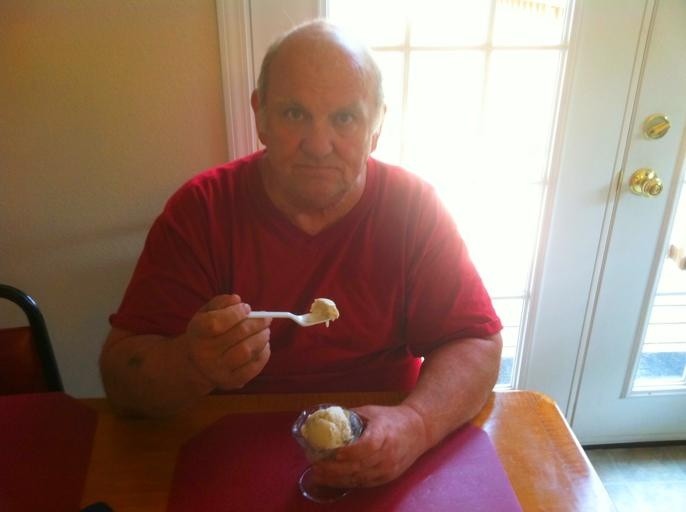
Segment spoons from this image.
[241,310,334,329]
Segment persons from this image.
[99,19,504,491]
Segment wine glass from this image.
[291,401,364,506]
